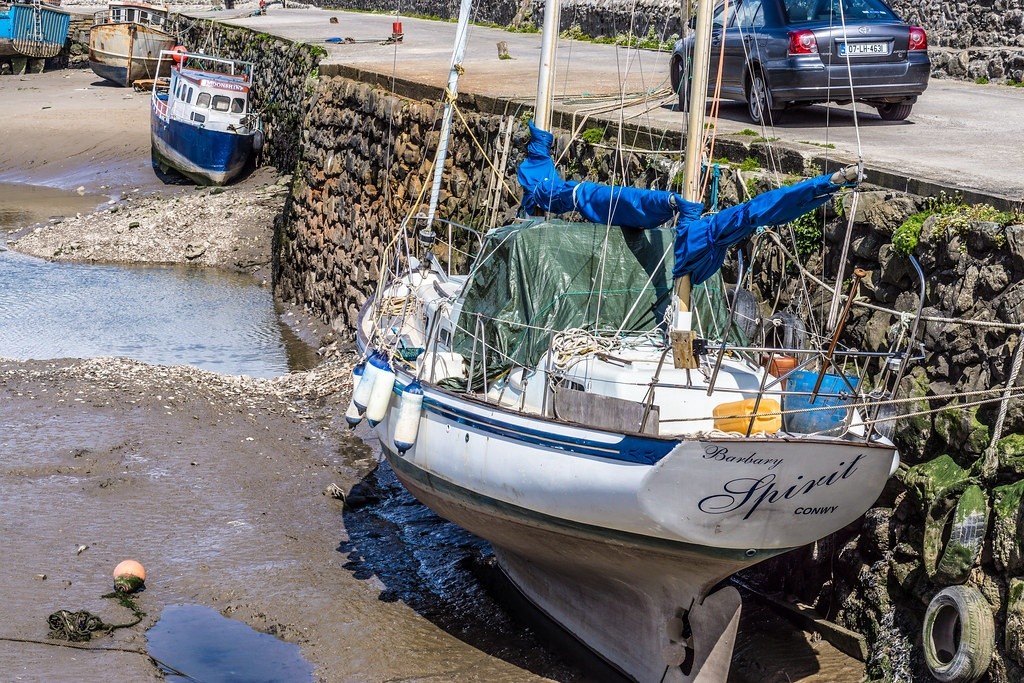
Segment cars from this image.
[670,0,931,126]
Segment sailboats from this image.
[353,0,926,683]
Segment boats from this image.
[88,0,178,87]
[151,47,265,185]
[0,0,71,75]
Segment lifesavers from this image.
[922,479,987,586]
[922,583,996,683]
[252,131,263,149]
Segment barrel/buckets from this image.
[784,370,861,440]
